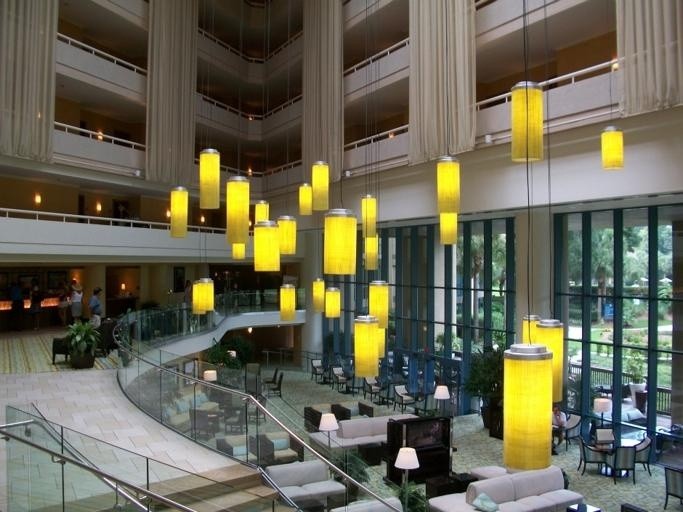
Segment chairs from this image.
[47,298,174,369]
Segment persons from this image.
[8,279,84,331]
[85,286,103,329]
[551,406,566,448]
[230,282,240,313]
[184,280,191,302]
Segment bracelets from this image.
[556,415,559,416]
